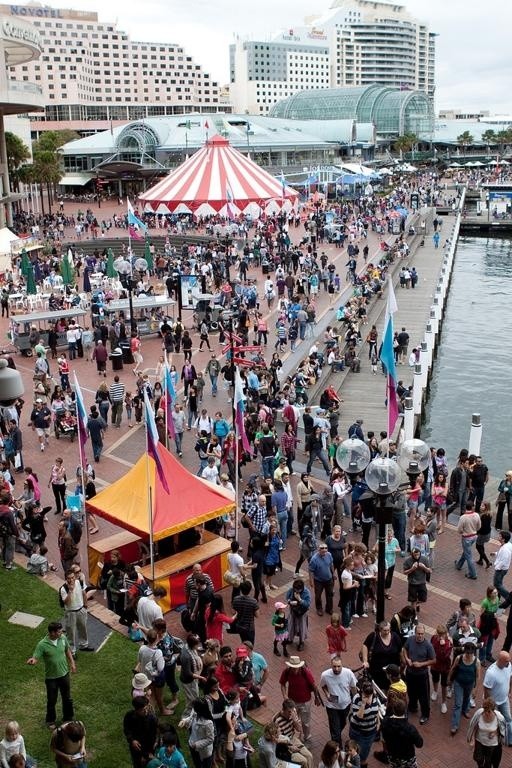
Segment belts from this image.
[68,606,86,613]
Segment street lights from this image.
[486,188,490,221]
[213,222,241,290]
[112,255,148,338]
[305,159,415,253]
[332,435,433,630]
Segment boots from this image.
[283,647,289,657]
[274,647,281,656]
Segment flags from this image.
[185,118,192,129]
[204,119,210,128]
[247,121,251,130]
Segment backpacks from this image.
[59,579,84,609]
[263,408,274,429]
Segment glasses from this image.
[320,547,328,550]
[76,568,81,576]
[303,472,308,475]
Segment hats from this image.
[383,664,400,677]
[316,407,326,414]
[464,642,476,654]
[131,673,153,690]
[284,656,306,669]
[236,644,247,658]
[274,602,287,610]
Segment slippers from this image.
[385,592,392,600]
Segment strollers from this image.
[53,407,77,441]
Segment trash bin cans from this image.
[118,339,134,364]
[109,351,123,370]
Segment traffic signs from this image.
[234,347,262,351]
[222,344,231,355]
[223,332,231,340]
[232,334,245,345]
[233,358,255,367]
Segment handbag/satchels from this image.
[168,633,184,666]
[96,396,102,404]
[223,569,244,589]
[358,631,376,663]
[241,506,258,528]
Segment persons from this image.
[2,163,511,767]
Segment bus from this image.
[7,292,241,359]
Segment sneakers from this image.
[293,571,304,579]
[419,717,427,724]
[345,594,377,631]
[454,559,492,579]
[244,745,254,754]
[432,691,438,701]
[470,698,476,707]
[270,584,278,590]
[447,687,453,698]
[440,703,448,714]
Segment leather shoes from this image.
[464,712,472,719]
[79,646,94,651]
[166,699,179,709]
[298,642,305,651]
[317,608,323,616]
[450,729,457,734]
[328,610,333,616]
